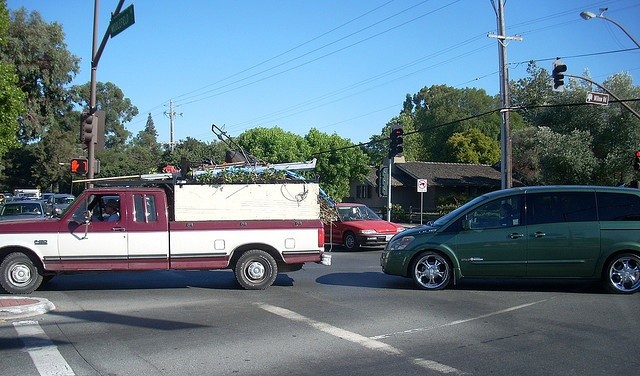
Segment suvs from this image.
[41,193,55,204]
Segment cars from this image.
[5,193,13,198]
[46,196,53,214]
[0,194,5,202]
[324,203,406,251]
[0,200,52,220]
[52,194,77,217]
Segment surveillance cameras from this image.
[598,5,610,18]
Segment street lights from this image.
[580,7,640,48]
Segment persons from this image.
[91,199,120,221]
[500,203,513,226]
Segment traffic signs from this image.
[417,179,428,192]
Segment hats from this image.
[104,199,120,209]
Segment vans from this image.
[380,185,640,294]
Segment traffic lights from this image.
[71,158,88,174]
[552,60,567,92]
[375,164,389,197]
[389,125,403,158]
[80,112,93,142]
[94,159,100,175]
[634,150,640,170]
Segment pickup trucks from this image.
[0,188,325,294]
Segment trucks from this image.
[13,193,26,200]
